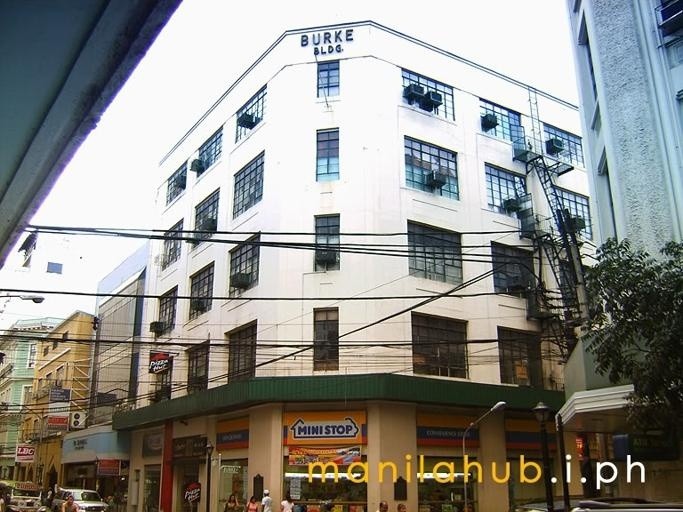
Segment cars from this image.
[51,489,109,512]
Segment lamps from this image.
[131,480,139,505]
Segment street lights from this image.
[12,401,43,479]
[205,442,214,512]
[0,294,46,304]
[463,400,508,512]
[533,399,553,511]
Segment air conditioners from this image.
[70,411,86,429]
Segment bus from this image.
[0,480,45,512]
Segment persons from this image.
[223,489,408,512]
[60,495,79,512]
[45,487,51,507]
